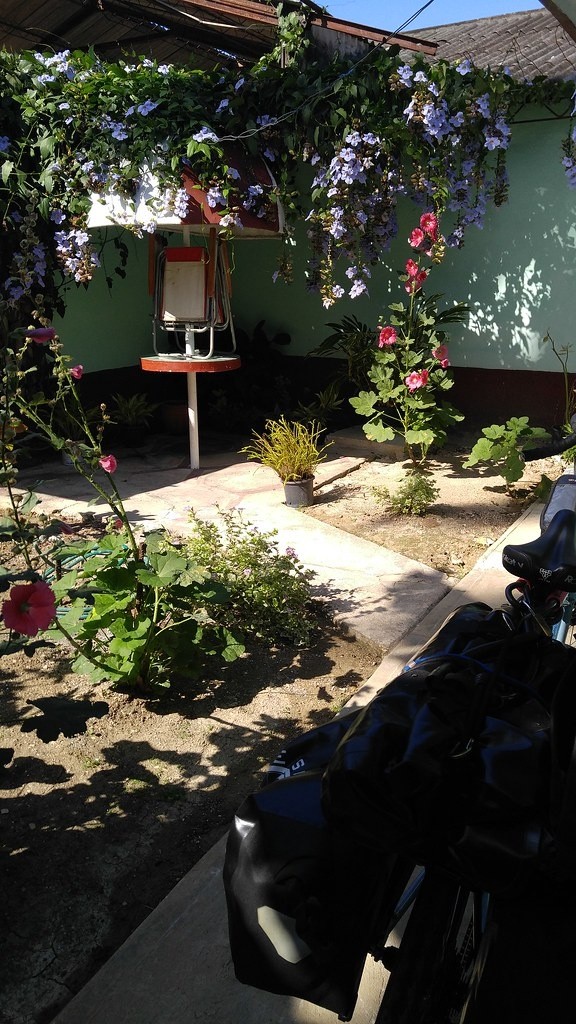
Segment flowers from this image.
[0,9,576,343]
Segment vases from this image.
[161,398,188,438]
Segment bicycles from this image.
[350,423,576,1024]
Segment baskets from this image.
[540,474,576,533]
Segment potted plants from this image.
[207,376,347,507]
[38,389,165,465]
